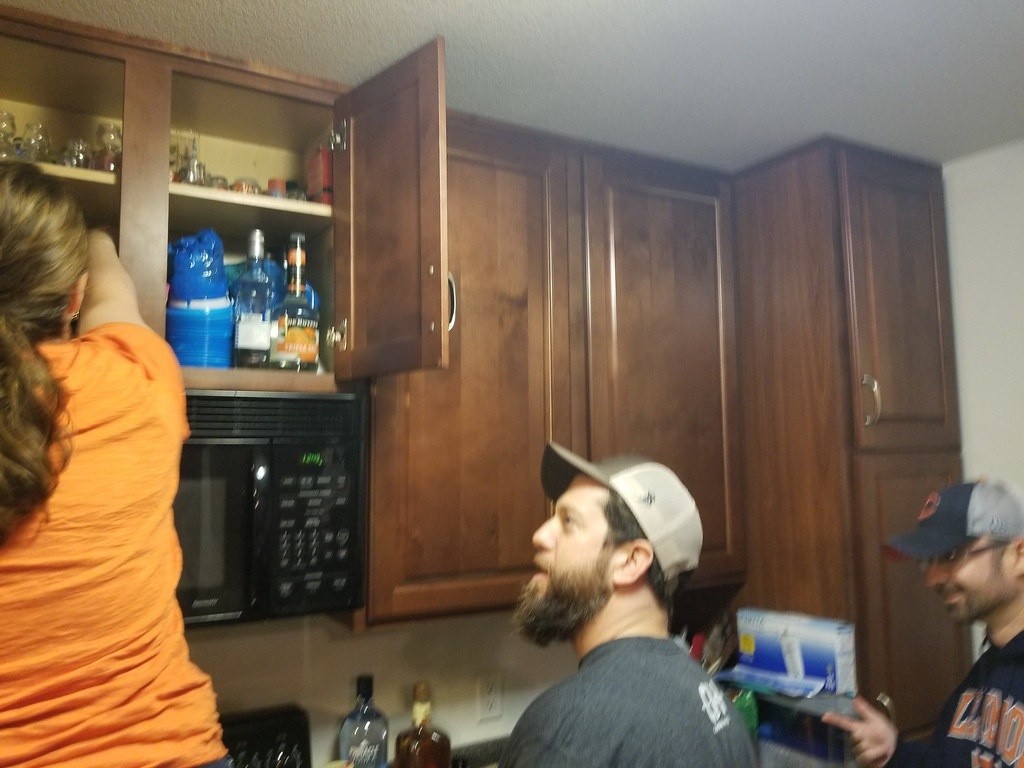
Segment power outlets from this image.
[476,674,503,720]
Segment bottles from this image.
[274,233,319,373]
[395,681,452,768]
[339,673,387,768]
[235,227,271,369]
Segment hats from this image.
[885,483,1024,560]
[539,436,703,581]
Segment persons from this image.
[822,477,1023,768]
[489,441,759,768]
[1,157,238,768]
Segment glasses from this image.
[916,542,1010,571]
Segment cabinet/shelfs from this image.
[324,107,745,636]
[0,4,457,392]
[670,134,975,746]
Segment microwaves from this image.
[174,389,370,628]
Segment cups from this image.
[170,123,299,198]
[0,109,122,172]
